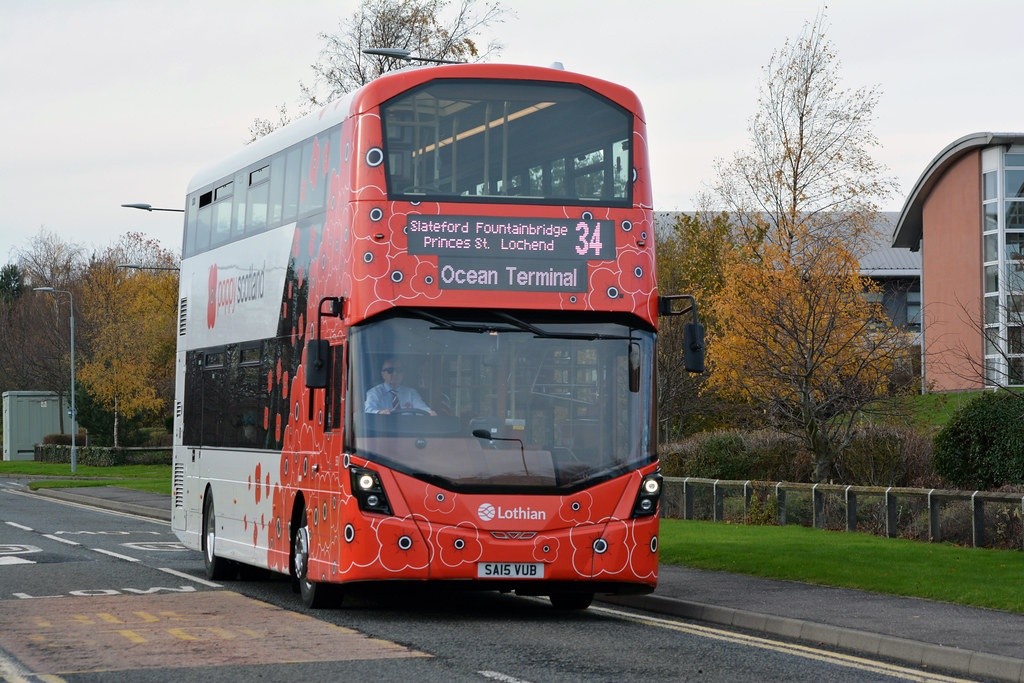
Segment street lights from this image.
[363,46,467,65]
[32,285,80,474]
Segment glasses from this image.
[383,367,404,374]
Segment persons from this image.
[364,360,439,416]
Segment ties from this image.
[390,390,401,414]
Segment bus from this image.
[169,60,708,612]
